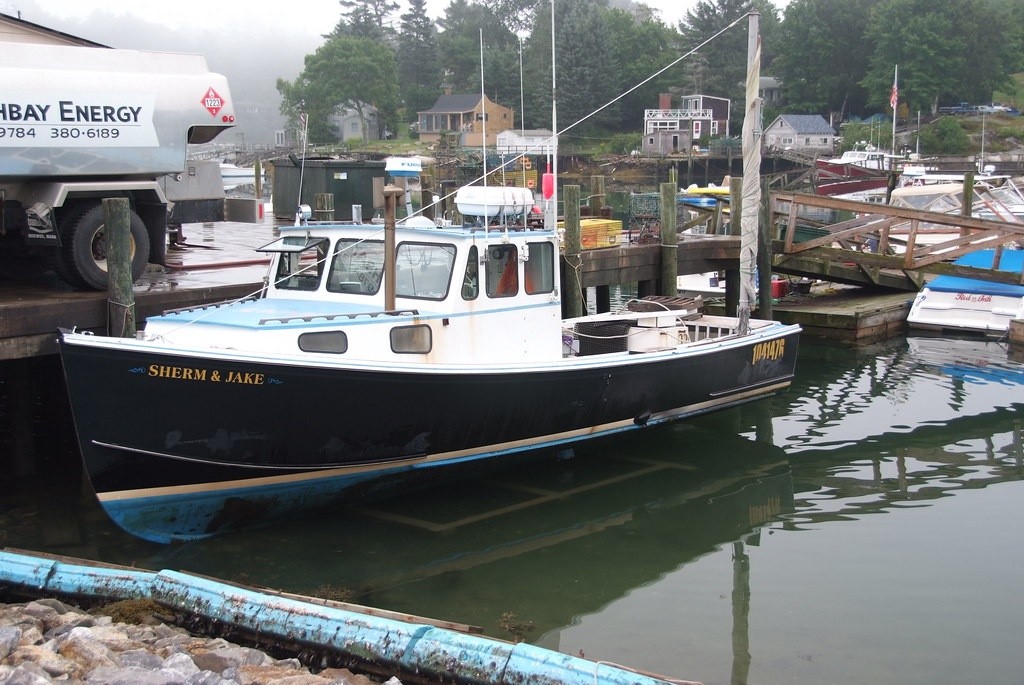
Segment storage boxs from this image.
[628,193,687,216]
[555,218,623,251]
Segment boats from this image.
[124,432,797,643]
[52,2,1024,546]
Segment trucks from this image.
[1,41,238,296]
